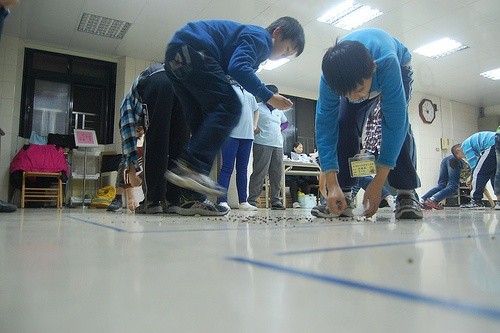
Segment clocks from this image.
[419,98,438,125]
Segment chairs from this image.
[20,141,65,210]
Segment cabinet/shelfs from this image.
[67,148,99,206]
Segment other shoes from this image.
[489,202,500,209]
[217,201,231,210]
[460,198,485,209]
[270,203,286,210]
[239,202,258,211]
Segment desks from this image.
[266,159,319,208]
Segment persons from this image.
[420,153,495,209]
[215,80,259,210]
[493,126,500,210]
[311,29,424,220]
[162,15,305,215]
[107,133,145,212]
[450,130,496,209]
[247,84,290,209]
[117,62,192,213]
[283,140,318,208]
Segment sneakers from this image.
[163,162,228,196]
[419,201,433,210]
[167,192,231,216]
[311,195,355,217]
[135,198,164,214]
[106,198,122,211]
[395,194,424,219]
[424,197,444,210]
